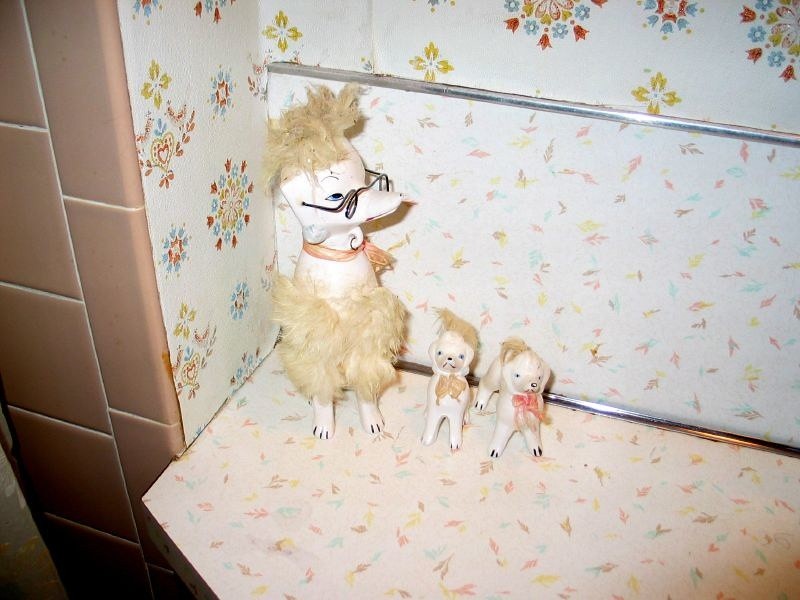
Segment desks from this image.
[136,357,800,600]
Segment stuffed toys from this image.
[413,313,481,451]
[473,336,551,458]
[262,87,404,439]
